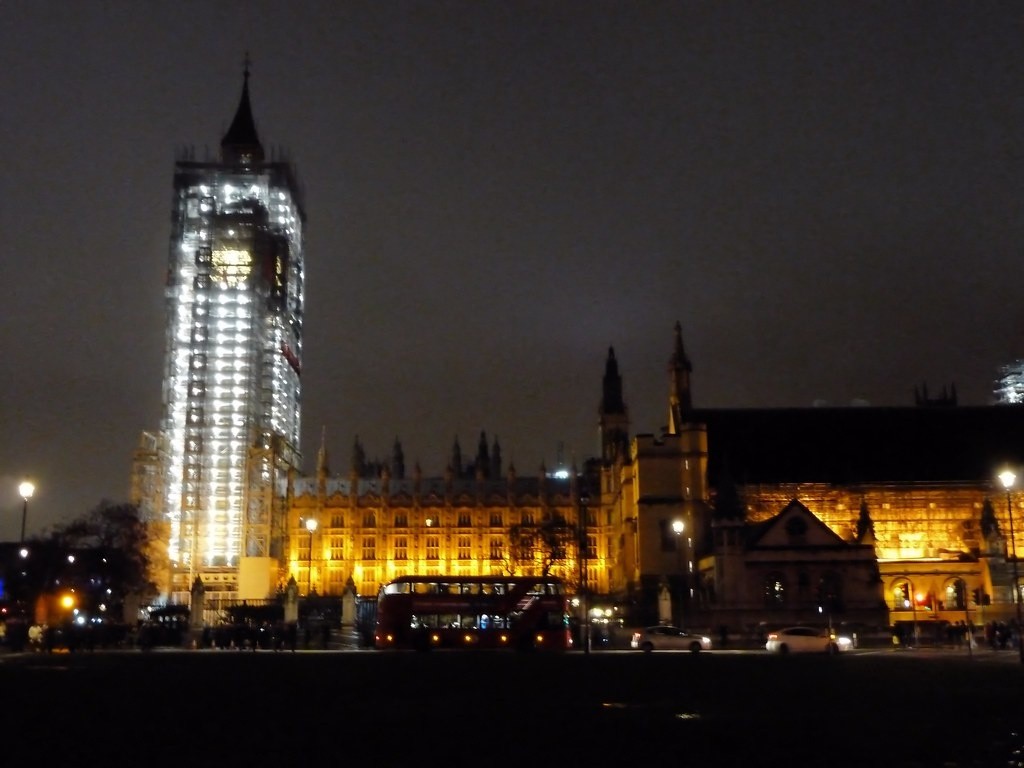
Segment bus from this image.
[374,575,571,653]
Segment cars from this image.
[630,626,712,653]
[764,627,853,654]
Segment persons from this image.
[0,615,333,654]
[890,620,1024,651]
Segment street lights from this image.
[305,518,318,597]
[889,576,919,651]
[17,480,34,588]
[553,440,589,652]
[998,469,1024,662]
[943,576,973,658]
